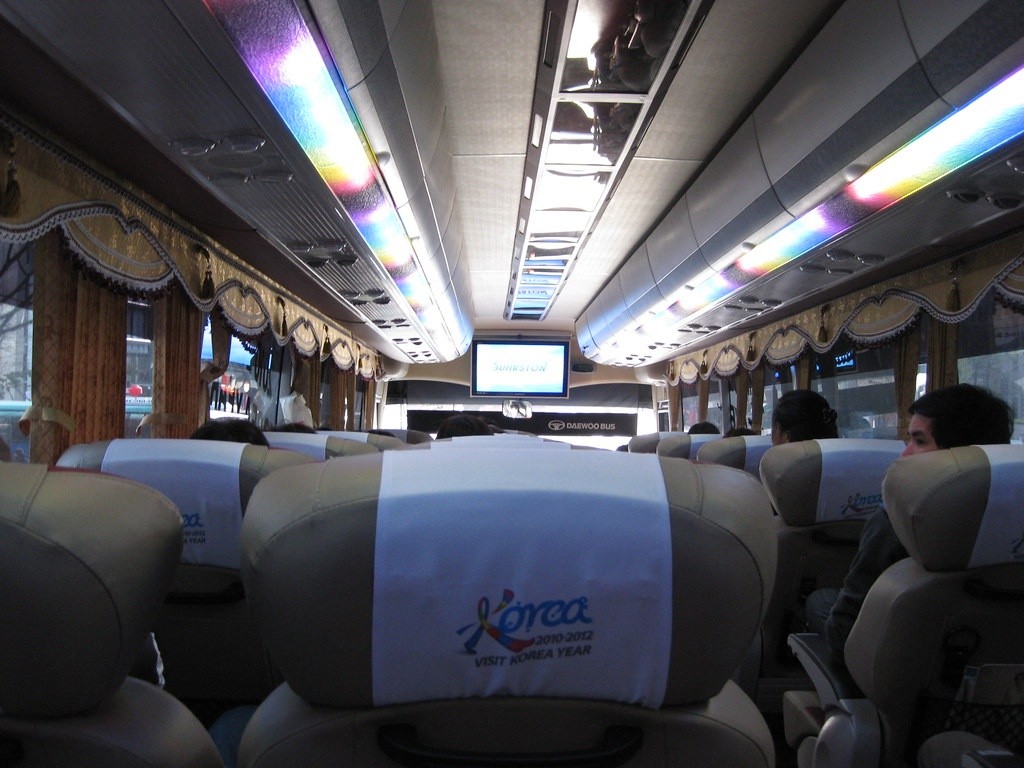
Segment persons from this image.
[805,382,1017,658]
[771,387,840,446]
[721,427,758,438]
[431,414,494,441]
[687,422,720,435]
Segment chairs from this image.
[696,434,773,484]
[263,430,381,460]
[629,432,669,456]
[777,444,1024,768]
[237,446,777,768]
[318,430,408,453]
[733,438,909,714]
[54,437,322,705]
[1,462,224,768]
[381,427,434,445]
[657,434,724,464]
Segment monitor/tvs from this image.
[470,338,570,399]
